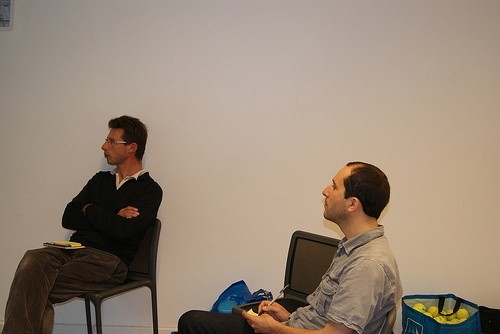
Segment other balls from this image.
[414,303,469,324]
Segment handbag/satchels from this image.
[211,280,274,315]
[402,294,481,334]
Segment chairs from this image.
[231,230,340,315]
[50,218,162,334]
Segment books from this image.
[43,240,85,249]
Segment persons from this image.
[177,162,397,334]
[2,115,163,334]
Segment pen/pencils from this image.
[257,284,291,316]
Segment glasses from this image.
[103,138,130,146]
